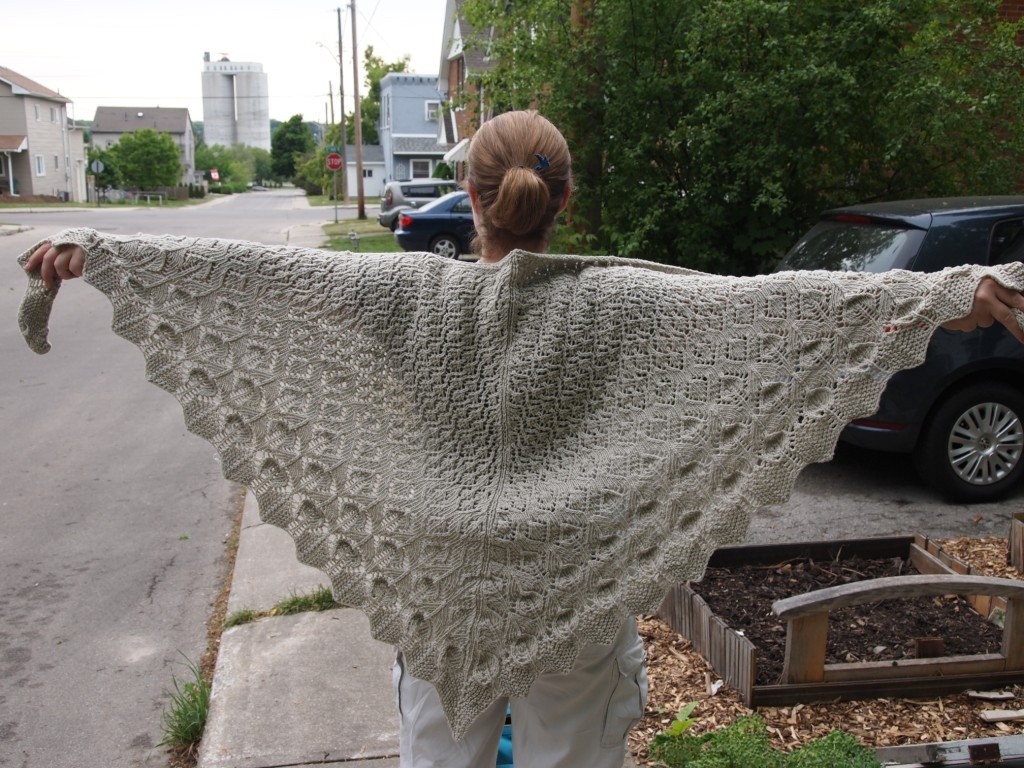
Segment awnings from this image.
[443,138,470,162]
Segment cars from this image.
[394,191,482,261]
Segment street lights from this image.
[314,41,349,206]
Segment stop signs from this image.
[325,153,343,171]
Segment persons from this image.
[18,109,1024,768]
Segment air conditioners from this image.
[428,108,438,121]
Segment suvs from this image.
[773,195,1024,502]
[377,176,473,233]
